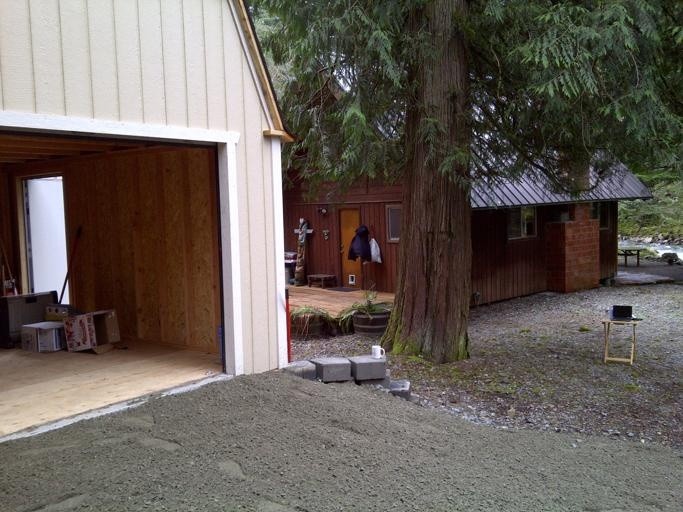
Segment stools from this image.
[307,273,337,289]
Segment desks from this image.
[599,313,643,366]
[617,246,640,267]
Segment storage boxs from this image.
[20,308,121,355]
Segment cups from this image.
[371,346,384,361]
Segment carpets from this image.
[326,286,361,293]
